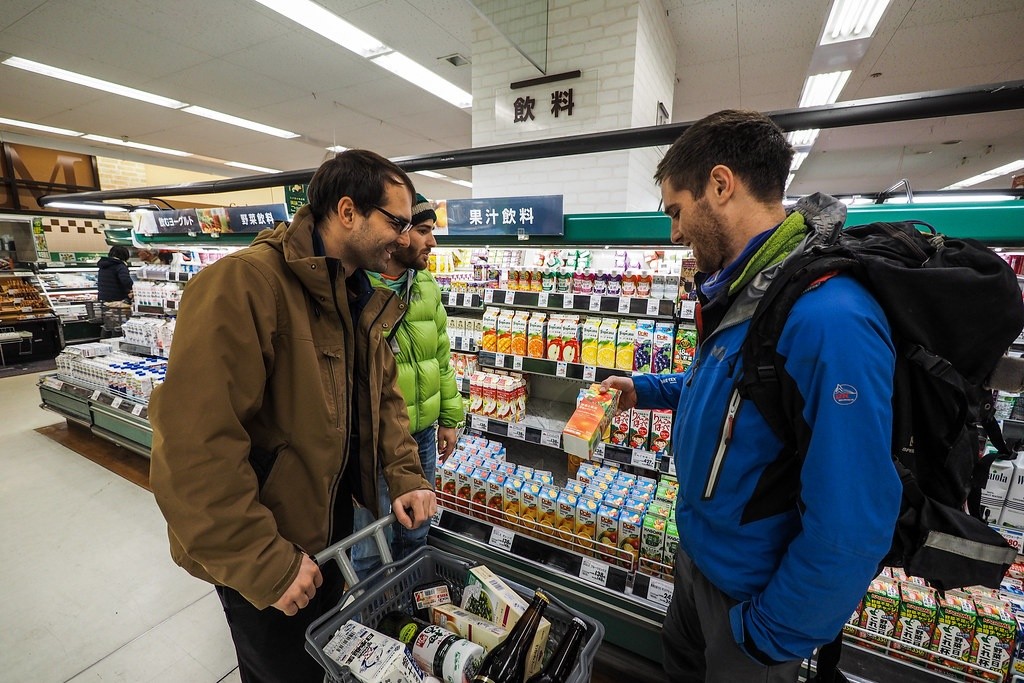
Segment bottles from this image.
[472,591,550,683]
[524,617,587,683]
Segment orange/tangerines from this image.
[511,337,544,358]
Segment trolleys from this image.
[304,507,605,683]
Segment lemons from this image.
[582,340,635,370]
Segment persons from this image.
[137,246,163,266]
[153,146,440,683]
[345,191,466,592]
[95,246,133,339]
[599,109,901,683]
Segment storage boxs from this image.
[321,563,551,683]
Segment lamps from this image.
[44,201,134,213]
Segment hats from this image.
[411,193,436,228]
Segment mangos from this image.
[482,335,511,353]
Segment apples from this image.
[547,338,579,363]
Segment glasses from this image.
[364,200,414,235]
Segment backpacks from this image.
[736,218,1024,590]
